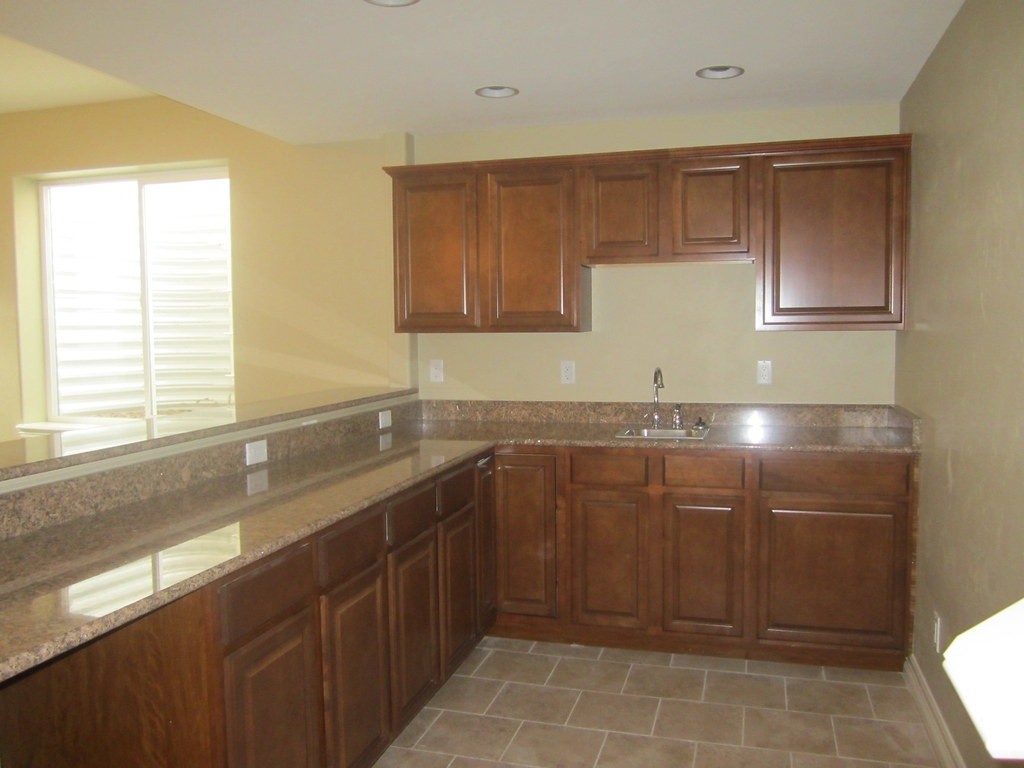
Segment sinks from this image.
[613,425,711,440]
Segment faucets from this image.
[649,366,667,430]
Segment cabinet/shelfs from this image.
[382,156,574,332]
[0,447,916,768]
[577,142,754,267]
[755,133,912,331]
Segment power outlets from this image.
[379,410,392,429]
[561,360,575,386]
[757,360,772,385]
[430,359,443,382]
[931,609,940,653]
[246,437,268,465]
